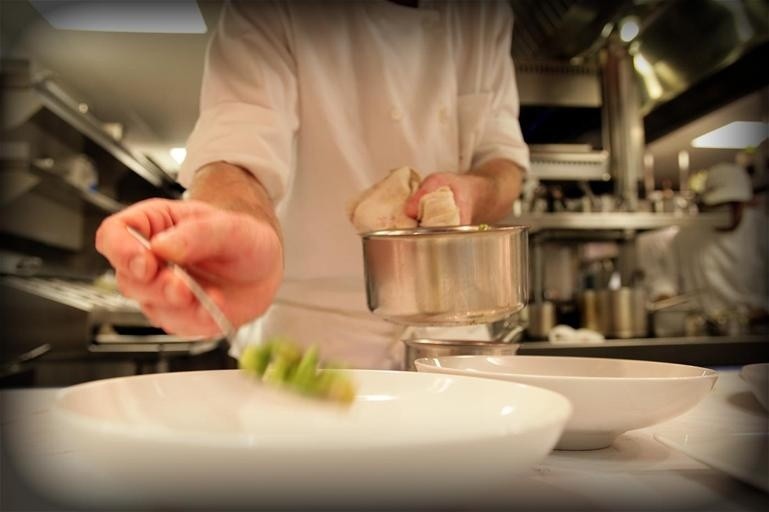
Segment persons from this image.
[93,0,531,379]
[634,163,768,314]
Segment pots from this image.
[580,283,700,341]
[355,166,531,329]
[401,322,524,374]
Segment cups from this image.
[524,301,556,343]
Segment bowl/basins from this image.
[415,354,721,451]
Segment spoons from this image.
[125,223,356,405]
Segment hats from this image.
[704,163,752,205]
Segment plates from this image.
[54,365,572,512]
[651,420,769,496]
